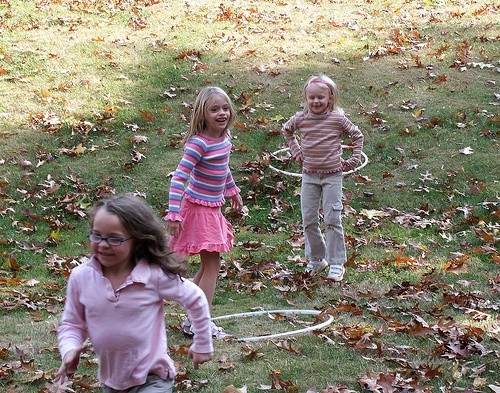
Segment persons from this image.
[53,193,214,393]
[163,86,244,337]
[281,74,364,282]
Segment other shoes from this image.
[189,326,230,340]
[182,319,193,337]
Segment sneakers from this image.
[306,259,328,275]
[328,264,345,281]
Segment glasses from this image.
[87,234,134,246]
